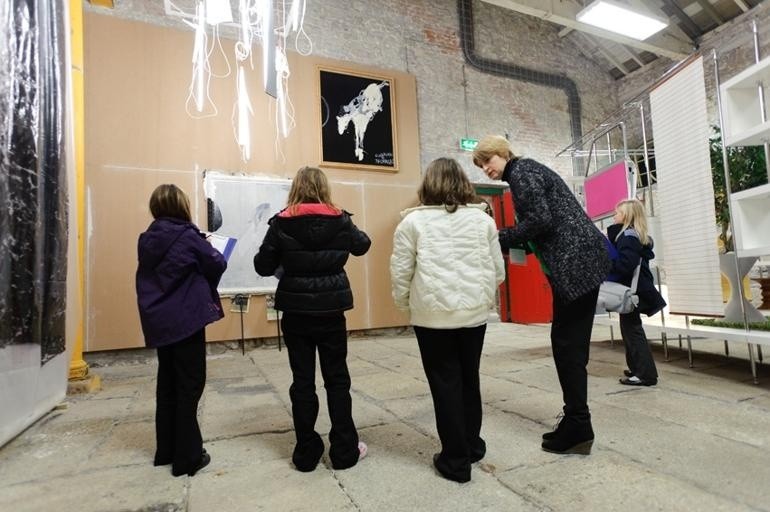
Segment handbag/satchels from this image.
[597,282,639,315]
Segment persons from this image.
[473,134,612,456]
[251,165,371,472]
[134,183,228,477]
[389,155,508,486]
[604,198,668,387]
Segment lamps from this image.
[576,0,670,41]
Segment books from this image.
[198,231,239,266]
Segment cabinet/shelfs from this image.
[719,55,770,258]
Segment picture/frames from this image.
[315,64,400,174]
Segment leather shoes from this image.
[623,369,634,377]
[619,377,658,385]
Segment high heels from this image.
[541,407,594,455]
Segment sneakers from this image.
[154,447,210,477]
[433,440,490,483]
[357,441,367,459]
